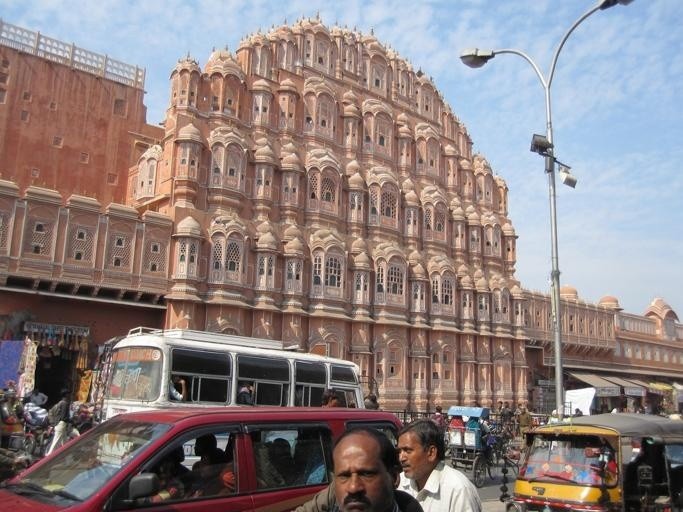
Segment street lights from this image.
[459,0,634,421]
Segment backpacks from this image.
[47,400,66,427]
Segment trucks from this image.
[515,412,682,512]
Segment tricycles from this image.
[0,392,36,455]
[447,405,501,469]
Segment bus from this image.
[91,324,380,477]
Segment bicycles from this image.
[472,429,506,488]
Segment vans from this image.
[0,406,404,511]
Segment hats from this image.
[61,389,72,396]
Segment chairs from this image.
[464,428,492,453]
[448,428,463,452]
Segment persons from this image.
[29,387,48,407]
[0,447,28,467]
[574,409,583,417]
[433,405,446,427]
[297,427,424,512]
[364,394,379,410]
[547,410,559,424]
[169,375,187,401]
[394,418,482,512]
[612,401,623,414]
[320,388,340,408]
[237,381,254,404]
[47,388,72,456]
[126,432,299,505]
[449,415,490,442]
[493,401,532,436]
